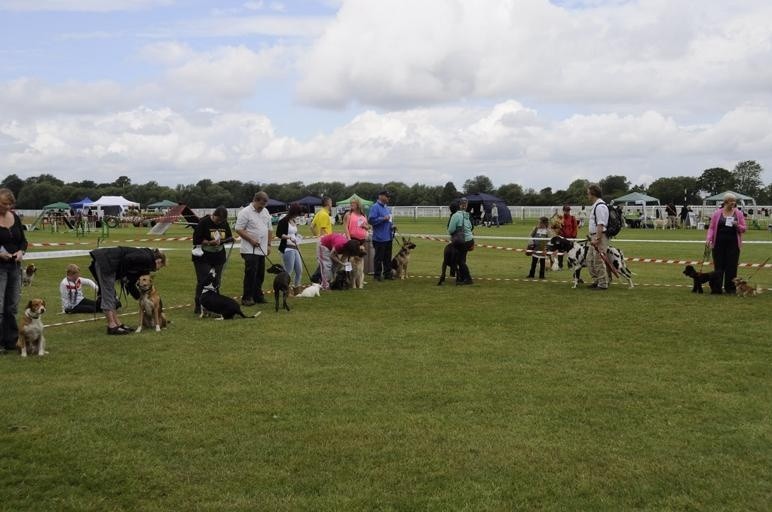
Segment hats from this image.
[563,205,571,210]
[379,190,394,197]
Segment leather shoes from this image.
[375,276,396,282]
[587,283,607,290]
[108,324,134,335]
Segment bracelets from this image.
[208,241,210,246]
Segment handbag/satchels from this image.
[524,243,536,256]
[450,226,465,245]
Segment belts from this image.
[590,232,605,235]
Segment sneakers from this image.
[457,278,472,283]
[241,298,272,305]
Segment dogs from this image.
[731,277,768,298]
[392,236,416,280]
[349,244,368,289]
[266,264,290,312]
[294,283,323,297]
[134,275,174,333]
[436,238,475,286]
[651,218,669,231]
[548,235,638,289]
[21,263,38,288]
[311,270,350,291]
[197,266,262,321]
[682,265,722,294]
[15,298,50,357]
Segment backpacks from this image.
[594,202,621,236]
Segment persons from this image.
[59,264,103,314]
[555,204,577,271]
[191,206,232,314]
[585,184,609,291]
[0,187,29,353]
[706,193,746,294]
[88,245,166,335]
[47,208,102,228]
[276,203,303,287]
[615,201,693,231]
[234,191,273,306]
[526,216,553,279]
[446,197,500,286]
[578,206,587,228]
[307,189,394,289]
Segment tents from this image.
[446,192,513,226]
[265,199,287,213]
[290,195,323,217]
